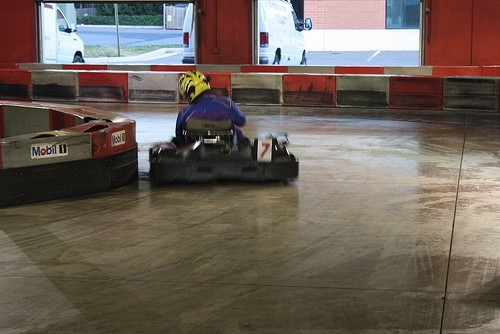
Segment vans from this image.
[40,3,86,64]
[180,1,312,68]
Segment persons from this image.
[171,68,253,152]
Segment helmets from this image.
[178,70,210,105]
